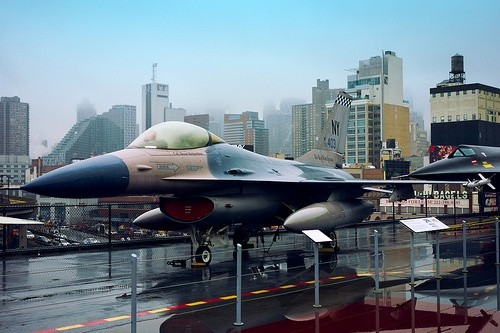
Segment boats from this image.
[410,262,499,309]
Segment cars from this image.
[25,220,187,245]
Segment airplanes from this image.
[407,144,500,191]
[18,88,477,269]
